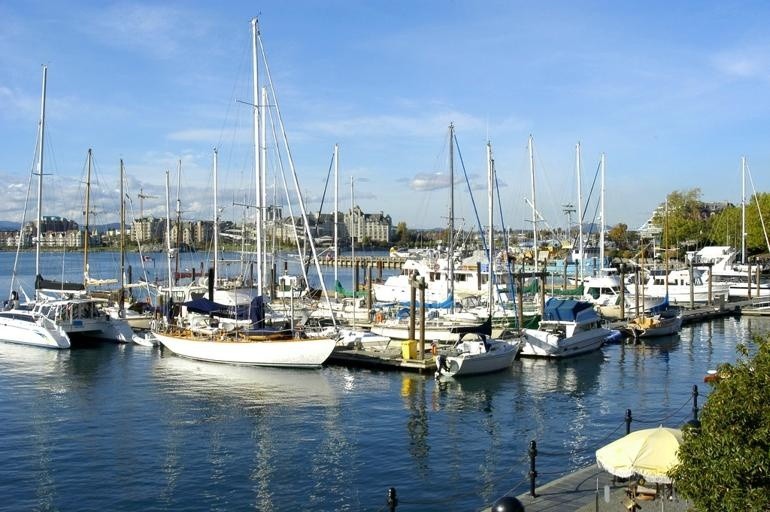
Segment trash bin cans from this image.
[400,340,417,359]
[375,312,383,322]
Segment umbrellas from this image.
[594,424,694,512]
